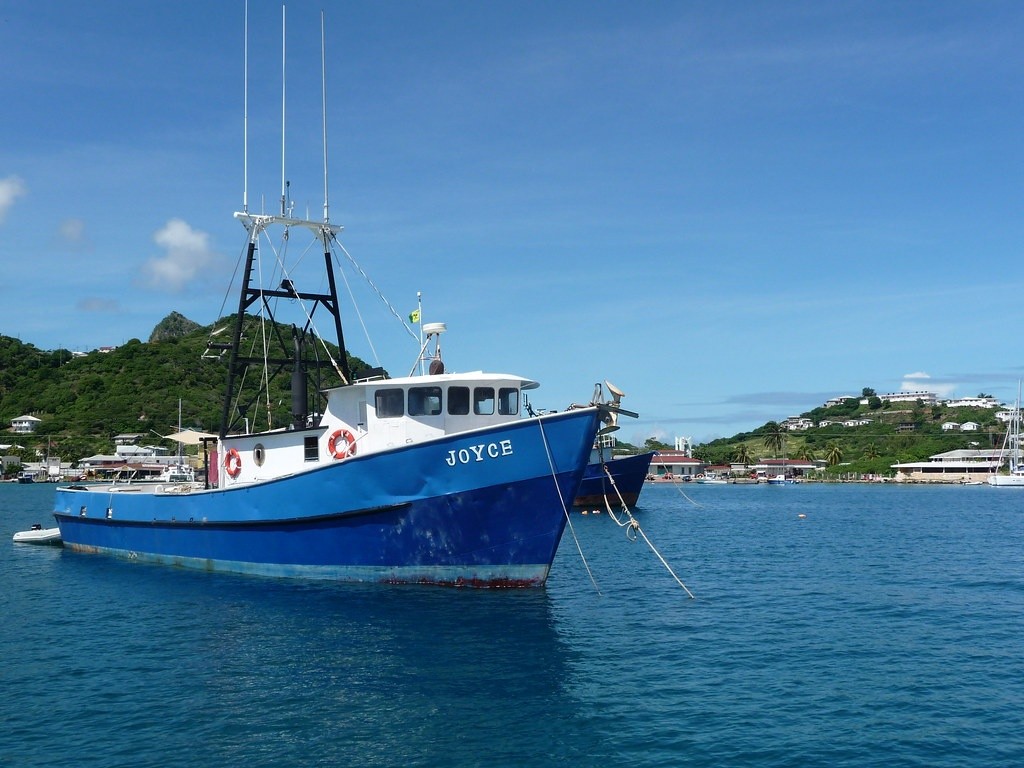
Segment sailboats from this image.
[987,378,1024,486]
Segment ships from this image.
[51,0,641,588]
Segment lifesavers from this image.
[327,429,356,459]
[224,449,241,476]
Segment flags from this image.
[409,309,420,323]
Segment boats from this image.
[571,424,657,511]
[12,526,60,542]
[695,477,728,485]
[16,471,34,485]
[766,474,794,484]
